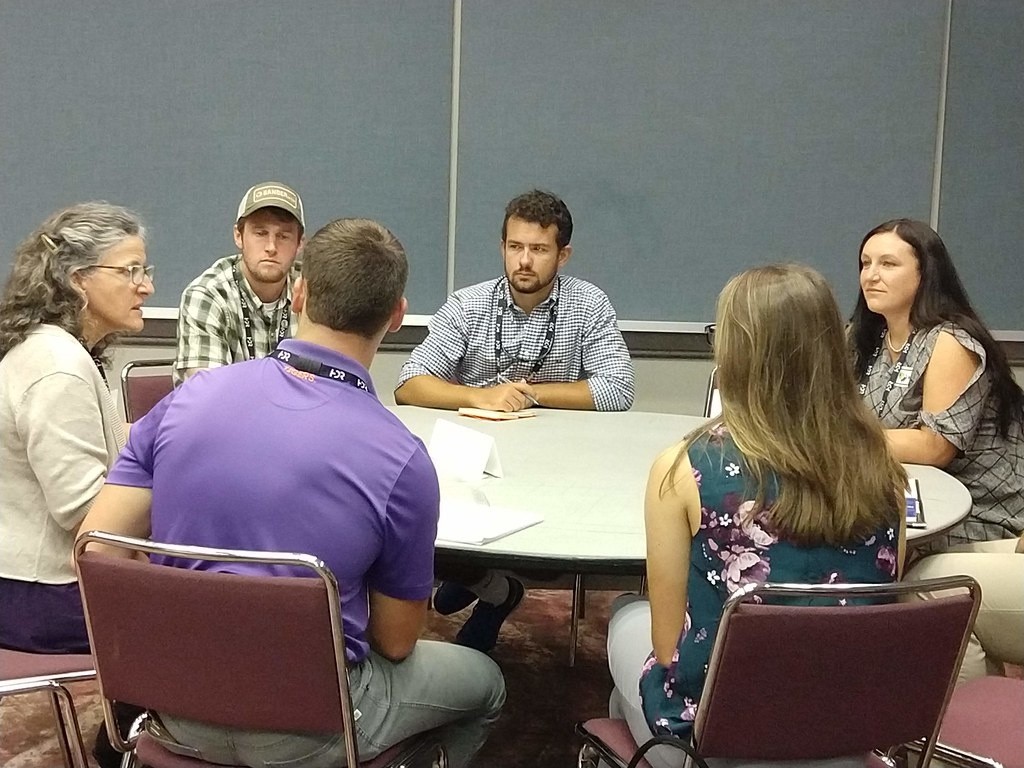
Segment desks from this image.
[384,403,973,669]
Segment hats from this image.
[235,181,306,228]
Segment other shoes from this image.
[454,575,526,655]
[433,579,478,616]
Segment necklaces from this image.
[886,331,911,353]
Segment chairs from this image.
[119,359,174,423]
[73,529,449,768]
[887,675,1024,768]
[0,650,96,768]
[573,574,982,768]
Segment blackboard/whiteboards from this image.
[0,0,1023,365]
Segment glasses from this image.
[704,324,716,347]
[77,264,155,286]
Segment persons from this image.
[172,180,307,391]
[392,190,636,651]
[899,533,1024,686]
[70,217,508,768]
[843,218,1024,570]
[577,263,912,768]
[0,201,156,768]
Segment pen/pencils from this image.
[497,373,541,407]
